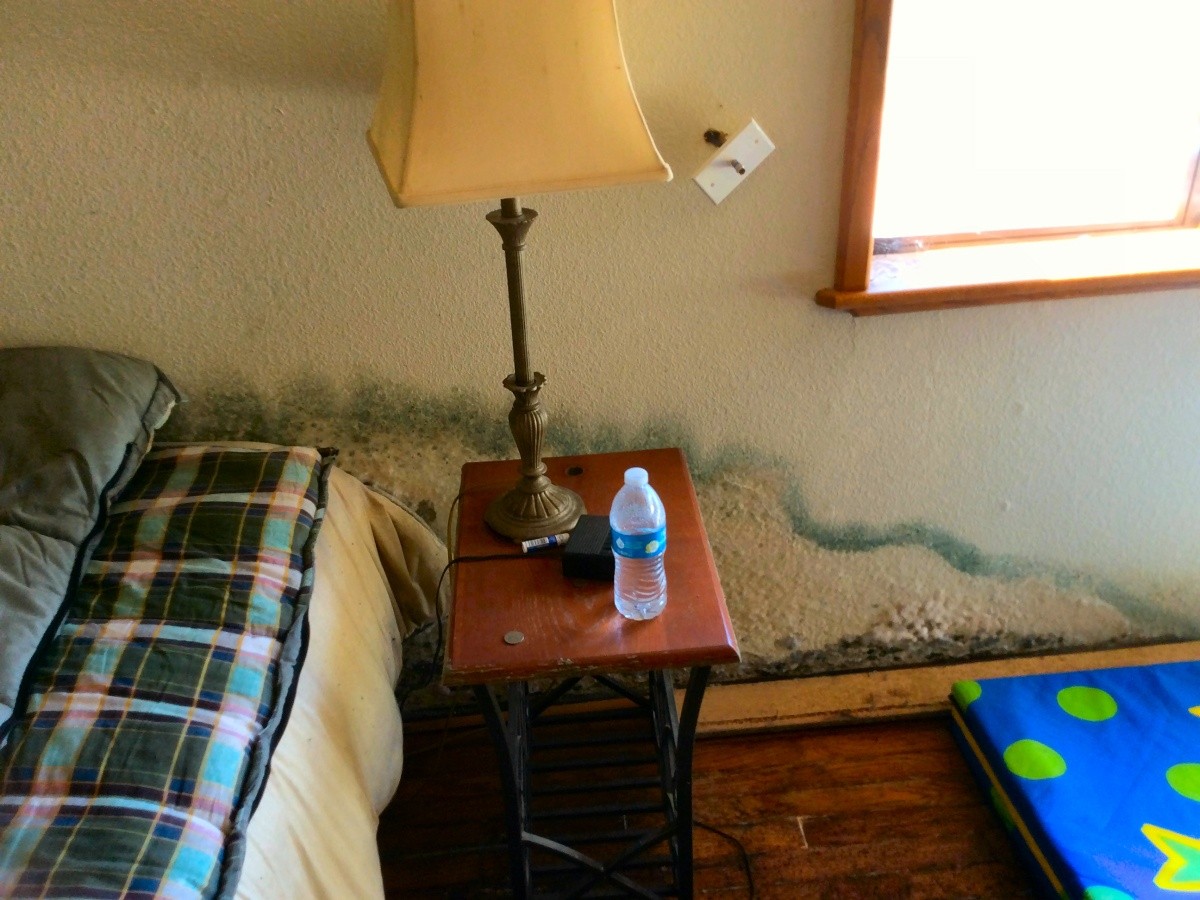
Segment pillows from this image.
[0,347,190,735]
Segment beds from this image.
[0,438,457,900]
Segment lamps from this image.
[364,0,676,543]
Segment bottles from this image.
[608,468,667,621]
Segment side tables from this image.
[439,445,745,900]
